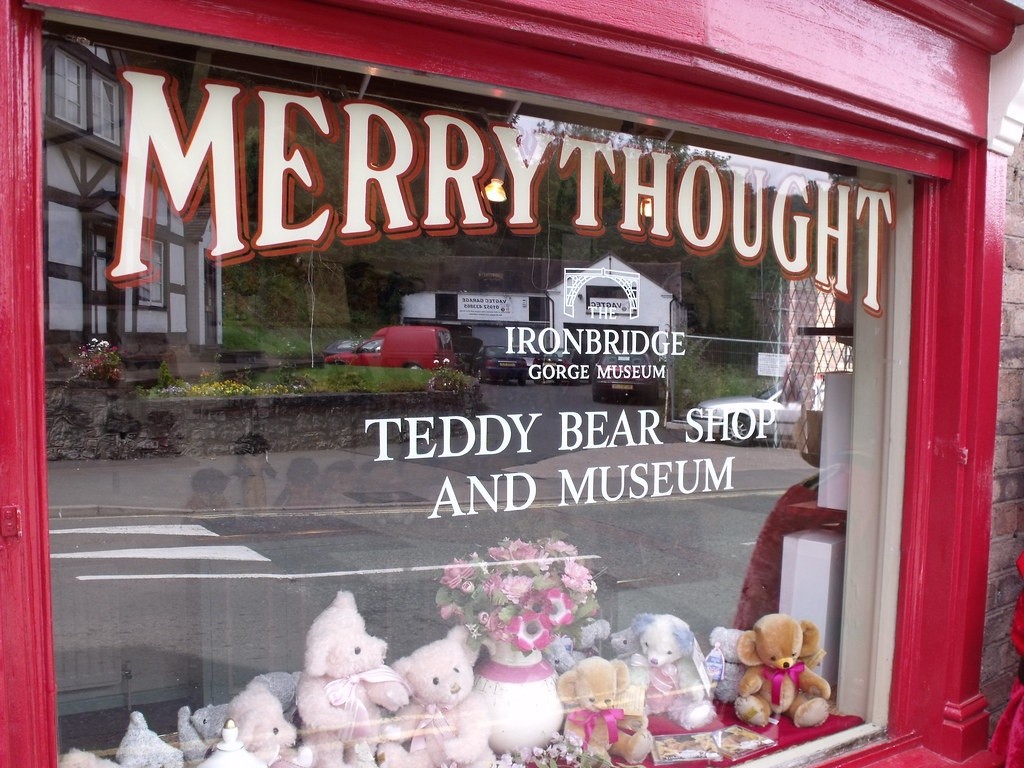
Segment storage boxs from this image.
[818,371,852,511]
[779,521,847,687]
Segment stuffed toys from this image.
[557,657,653,768]
[736,614,831,727]
[58,591,747,768]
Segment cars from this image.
[691,375,829,447]
[465,346,528,386]
[589,349,659,404]
[323,338,361,358]
[531,344,592,387]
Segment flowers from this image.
[435,531,600,655]
[69,338,128,381]
[490,732,646,768]
[429,358,464,395]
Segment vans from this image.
[325,325,456,378]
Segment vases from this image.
[472,635,566,756]
[87,363,118,379]
[437,379,459,392]
